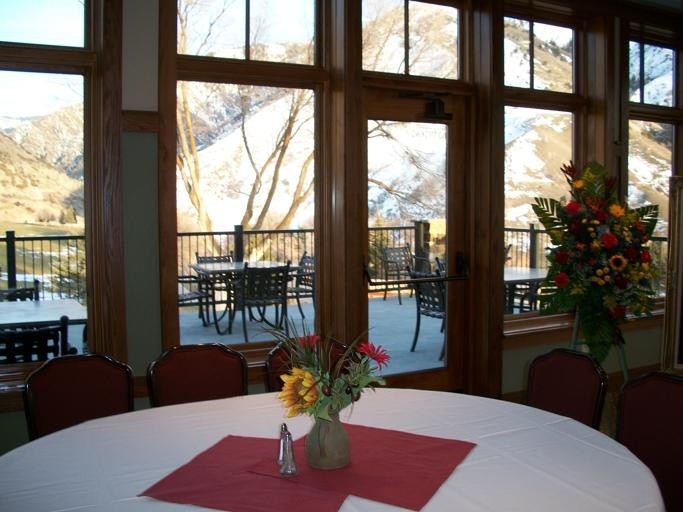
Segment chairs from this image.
[0,280,40,302]
[527,348,608,431]
[615,372,683,512]
[23,354,135,442]
[264,336,349,393]
[146,341,248,409]
[179,249,315,343]
[377,244,561,362]
[0,315,77,364]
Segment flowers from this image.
[251,314,391,423]
[532,157,658,364]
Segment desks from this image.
[0,299,88,330]
[0,388,666,512]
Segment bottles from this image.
[279,432,299,475]
[275,422,290,465]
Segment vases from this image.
[305,415,350,471]
